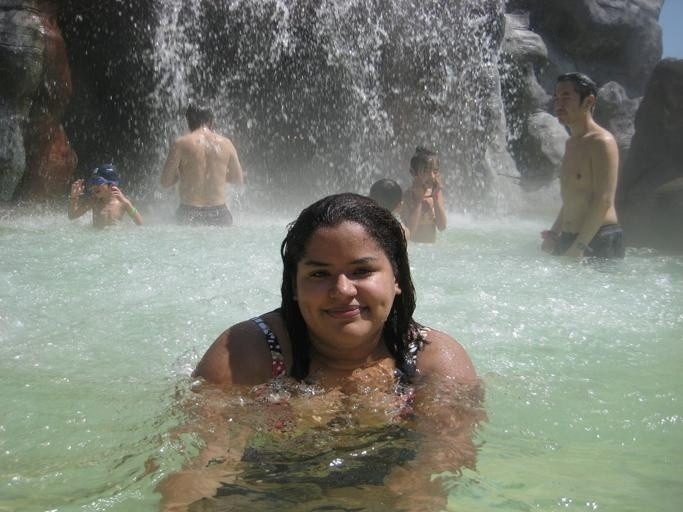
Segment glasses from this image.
[85,177,120,187]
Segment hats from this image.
[92,164,119,183]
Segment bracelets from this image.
[572,237,588,251]
[128,207,139,219]
[539,229,562,243]
[431,192,440,200]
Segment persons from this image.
[398,144,449,246]
[67,162,145,228]
[159,99,244,226]
[370,175,411,244]
[536,70,627,264]
[153,192,484,512]
